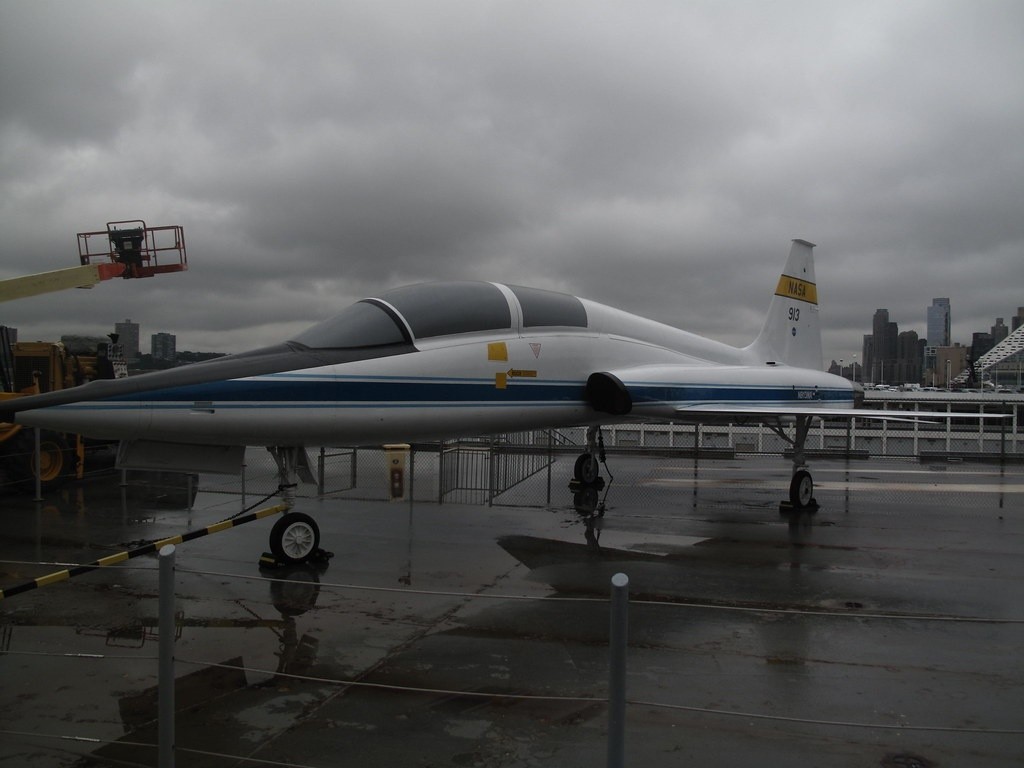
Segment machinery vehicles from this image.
[0,219,191,493]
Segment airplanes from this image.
[0,237,865,563]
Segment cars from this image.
[862,382,1024,392]
